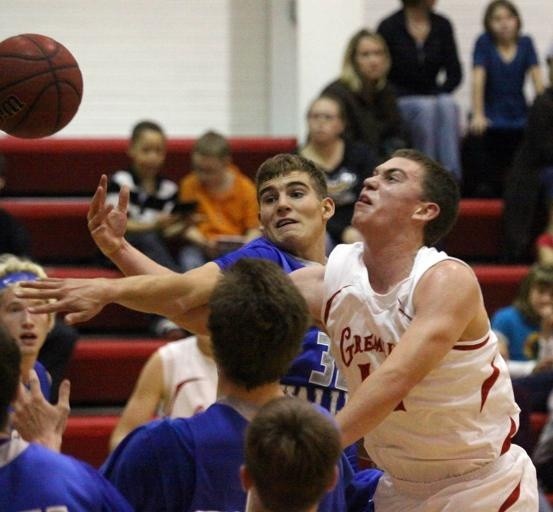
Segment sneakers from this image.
[157,319,187,340]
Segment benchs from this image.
[1,134,553,512]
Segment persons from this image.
[0,122,553,512]
[297,1,553,268]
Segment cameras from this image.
[170,203,197,215]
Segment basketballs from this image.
[0,35,83,137]
